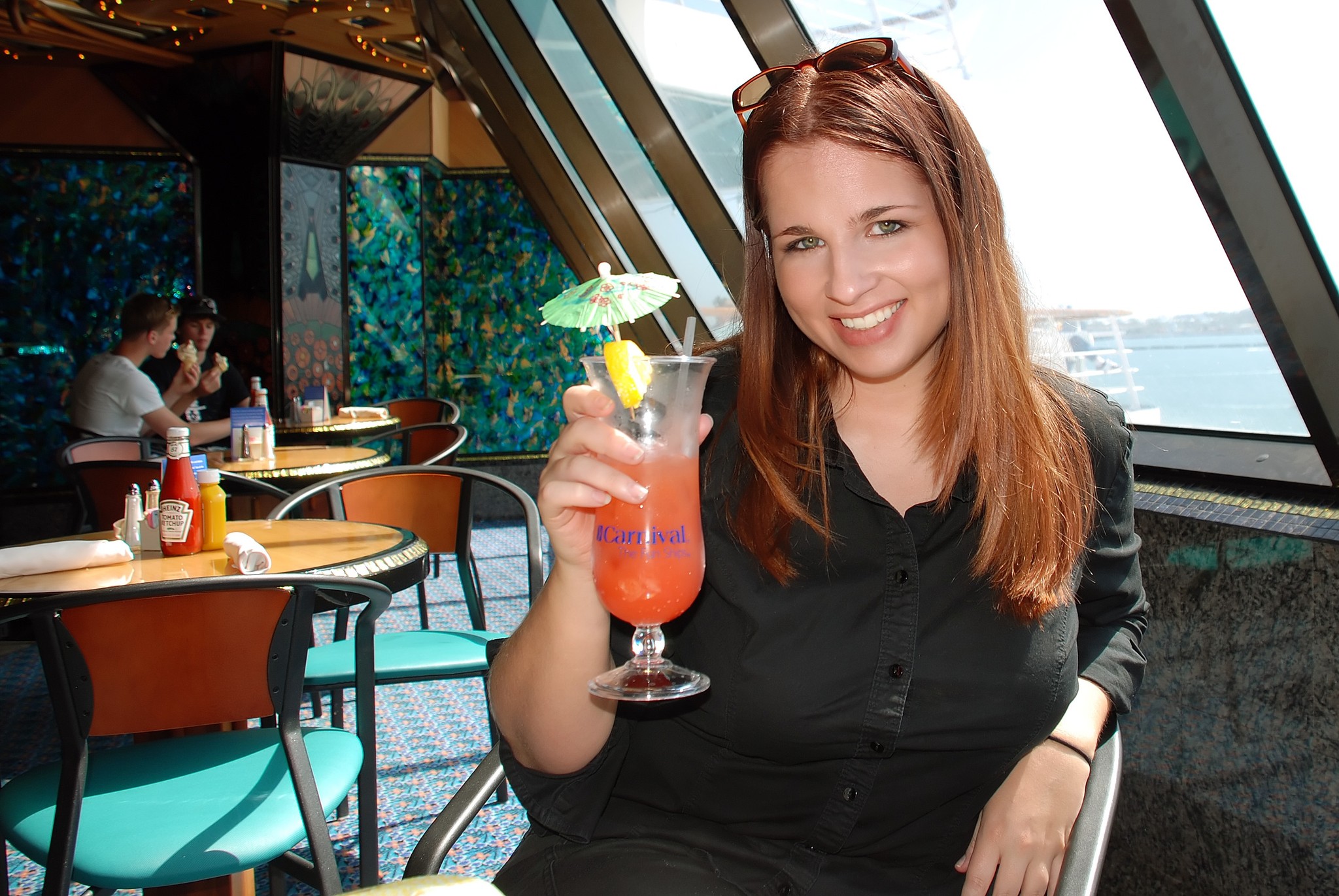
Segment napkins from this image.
[0,539,134,579]
[224,532,272,575]
[338,406,388,418]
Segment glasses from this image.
[152,303,179,327]
[732,38,942,137]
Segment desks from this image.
[273,414,402,441]
[191,445,393,522]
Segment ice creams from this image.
[213,352,228,373]
[176,340,199,372]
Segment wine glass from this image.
[581,352,714,703]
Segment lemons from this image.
[603,340,653,411]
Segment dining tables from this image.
[0,520,430,896]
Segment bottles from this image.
[239,423,253,461]
[297,397,302,423]
[249,376,263,407]
[157,427,203,556]
[260,423,276,461]
[197,467,227,551]
[145,478,160,511]
[290,397,300,423]
[124,483,144,550]
[253,388,274,425]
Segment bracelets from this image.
[1047,733,1093,768]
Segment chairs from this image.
[0,398,1125,896]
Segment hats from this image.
[177,295,221,325]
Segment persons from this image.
[487,40,1149,896]
[64,293,254,528]
[143,294,250,449]
